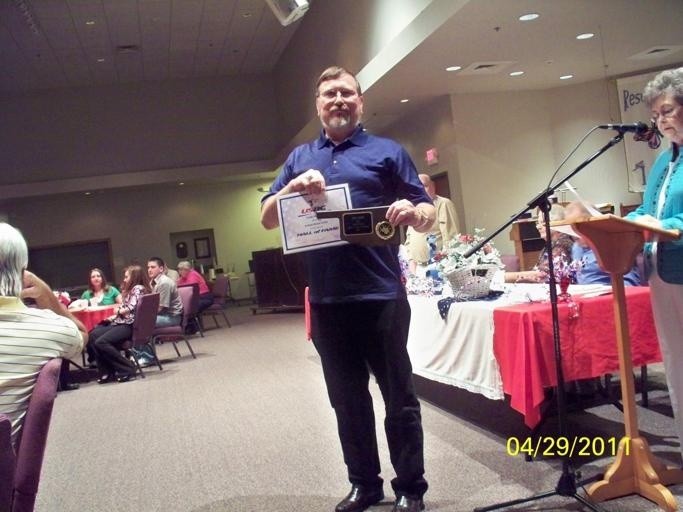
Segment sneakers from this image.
[59,356,156,389]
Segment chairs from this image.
[116,292,162,379]
[0,357,62,512]
[198,276,231,331]
[151,283,204,359]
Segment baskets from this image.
[445,263,497,300]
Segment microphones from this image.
[597,122,648,135]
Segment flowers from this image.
[431,227,506,272]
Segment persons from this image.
[572,200,640,286]
[0,221,89,457]
[619,69,683,459]
[505,203,576,284]
[260,66,436,511]
[399,174,460,263]
[56,257,213,391]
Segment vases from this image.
[446,264,493,301]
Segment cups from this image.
[90,297,98,307]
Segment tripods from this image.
[474,218,606,512]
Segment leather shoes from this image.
[336,486,384,512]
[391,496,424,512]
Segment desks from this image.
[306,284,664,462]
[67,305,120,379]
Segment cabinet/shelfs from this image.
[508,204,614,271]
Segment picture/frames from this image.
[194,237,211,259]
